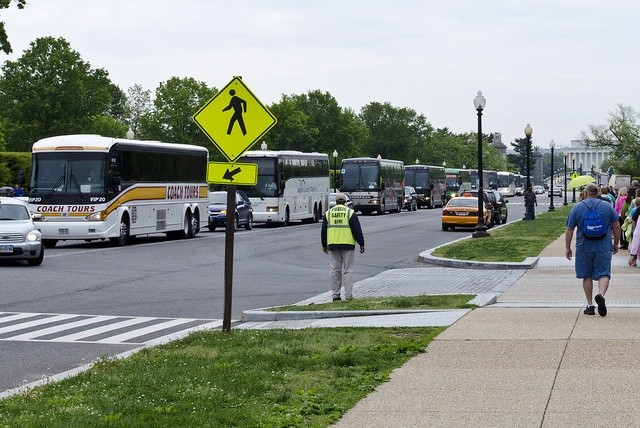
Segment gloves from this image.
[323,246,329,254]
[360,246,365,253]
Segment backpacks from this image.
[577,201,610,240]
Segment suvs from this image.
[459,189,504,226]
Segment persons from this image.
[524,187,537,220]
[565,183,621,317]
[621,180,640,269]
[321,193,365,302]
[598,183,621,211]
[0,184,25,197]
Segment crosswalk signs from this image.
[191,79,278,164]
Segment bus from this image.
[28,134,210,247]
[404,165,447,208]
[445,189,462,197]
[445,168,498,189]
[339,157,405,216]
[498,172,532,196]
[236,150,330,227]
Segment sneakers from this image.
[595,294,607,317]
[333,297,341,301]
[584,305,595,315]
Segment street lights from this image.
[590,162,602,186]
[563,149,569,206]
[333,150,338,193]
[548,139,555,212]
[572,154,576,202]
[522,124,534,220]
[579,160,583,192]
[472,91,491,238]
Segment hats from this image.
[336,194,346,202]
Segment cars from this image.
[208,191,253,232]
[441,197,492,230]
[330,193,354,210]
[548,187,562,197]
[493,191,509,224]
[0,197,46,266]
[534,185,545,194]
[404,186,417,211]
